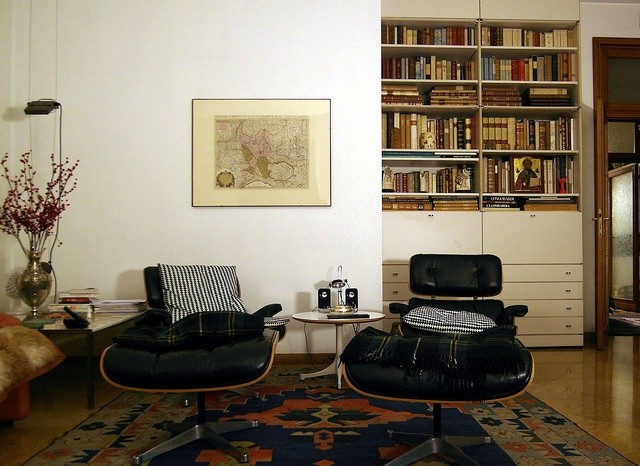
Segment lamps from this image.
[24,96,57,115]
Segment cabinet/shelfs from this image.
[483,211,583,348]
[381,17,582,211]
[382,209,483,332]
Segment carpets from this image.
[19,367,640,466]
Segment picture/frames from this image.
[191,99,331,207]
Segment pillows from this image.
[401,306,496,335]
[158,263,248,323]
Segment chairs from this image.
[0,313,64,434]
[135,267,286,343]
[390,254,529,337]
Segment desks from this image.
[10,313,144,409]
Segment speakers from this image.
[318,288,331,309]
[345,288,359,307]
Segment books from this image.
[481,25,578,47]
[381,24,476,44]
[481,196,578,210]
[482,83,572,107]
[47,287,148,315]
[483,157,574,193]
[381,84,478,106]
[381,54,475,80]
[394,164,475,193]
[382,194,479,212]
[481,53,576,81]
[382,111,475,149]
[482,114,574,150]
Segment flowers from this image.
[0,150,79,253]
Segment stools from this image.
[100,330,280,466]
[340,329,531,465]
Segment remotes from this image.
[326,314,371,319]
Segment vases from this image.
[16,253,52,320]
